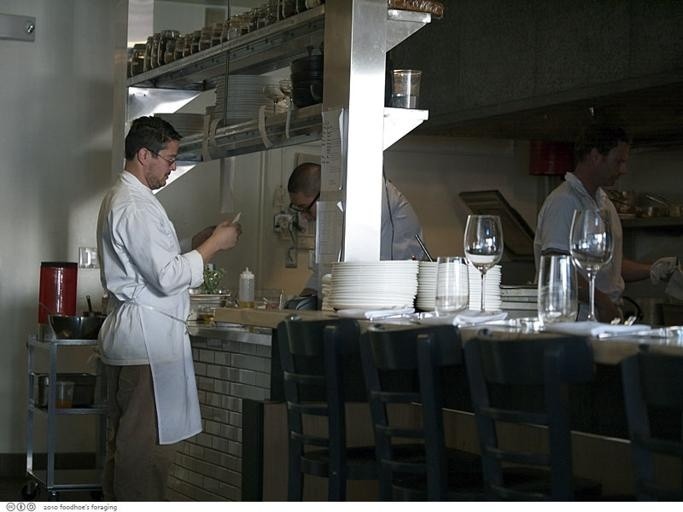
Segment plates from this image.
[152,73,293,140]
[52,339,98,346]
[330,259,503,314]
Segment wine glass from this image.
[464,216,504,319]
[568,209,615,322]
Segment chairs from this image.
[274,317,682,502]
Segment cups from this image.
[391,69,424,109]
[436,255,468,320]
[538,256,578,327]
[196,306,215,326]
[57,381,75,409]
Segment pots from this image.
[38,295,106,339]
[600,188,683,218]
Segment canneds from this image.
[127,0,323,78]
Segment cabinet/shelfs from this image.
[111,0,433,186]
[19,338,113,500]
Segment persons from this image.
[96,116,241,501]
[534,122,680,326]
[287,162,424,296]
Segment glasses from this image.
[144,146,176,166]
[289,192,320,214]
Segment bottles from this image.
[238,266,255,308]
[129,0,326,82]
